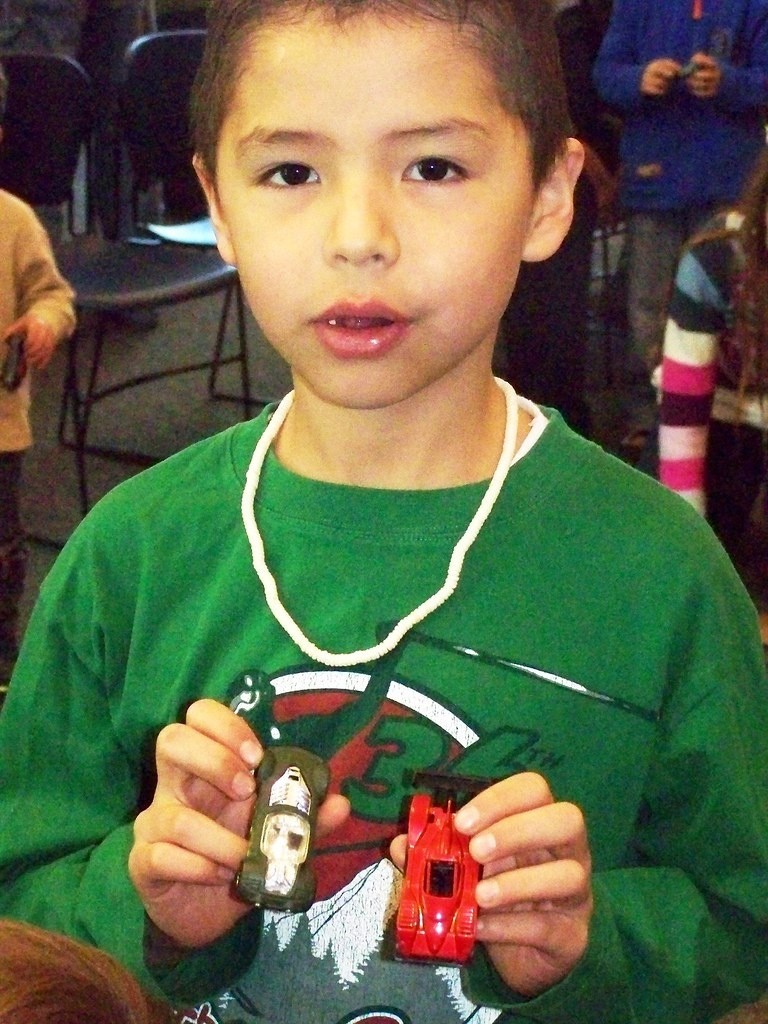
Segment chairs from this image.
[0,30,276,555]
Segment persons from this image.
[0,915,176,1024]
[651,124,768,663]
[589,0,768,463]
[0,63,77,709]
[0,0,768,1024]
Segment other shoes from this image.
[620,424,656,453]
